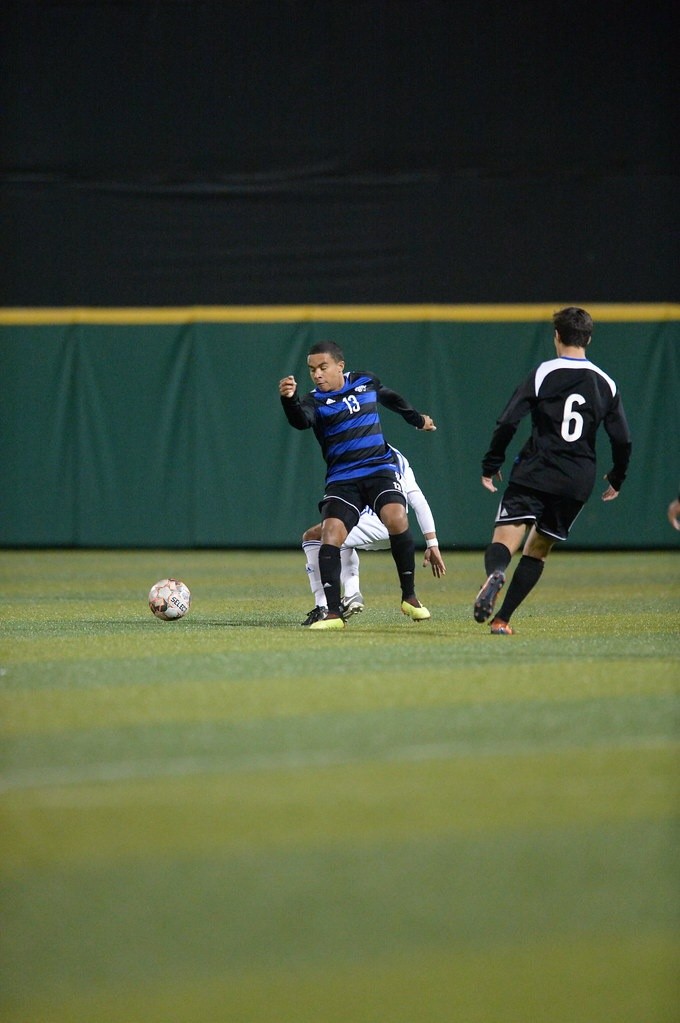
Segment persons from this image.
[473,305,632,634]
[300,440,448,630]
[278,340,436,627]
[668,494,680,532]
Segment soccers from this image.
[148,579,191,622]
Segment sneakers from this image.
[474,569,506,624]
[301,604,329,626]
[490,617,515,635]
[308,618,344,630]
[339,592,365,622]
[401,600,431,622]
